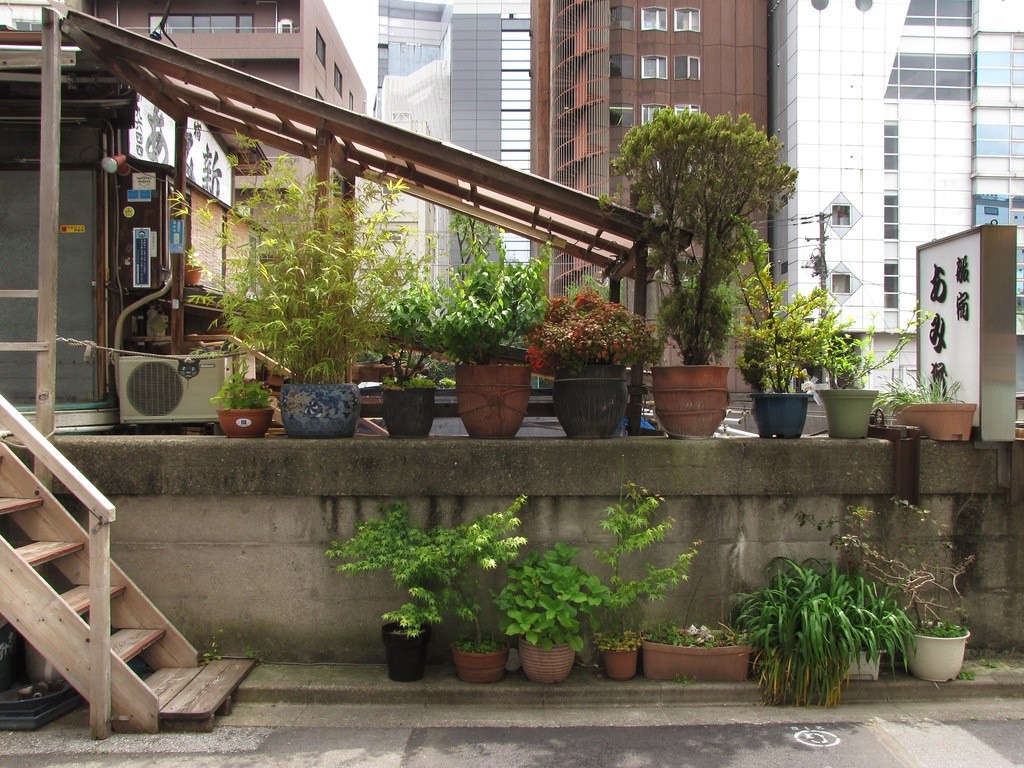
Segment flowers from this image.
[531,283,653,369]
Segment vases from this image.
[554,368,628,439]
[184,264,203,286]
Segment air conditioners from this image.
[324,493,527,683]
[118,353,232,423]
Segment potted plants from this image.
[876,368,977,442]
[734,215,815,438]
[167,127,410,438]
[210,365,275,439]
[639,624,751,682]
[382,295,436,439]
[814,321,880,438]
[908,620,971,682]
[651,287,732,438]
[489,540,608,682]
[381,603,433,682]
[832,570,915,681]
[595,627,639,680]
[437,208,532,438]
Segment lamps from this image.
[101,154,127,174]
